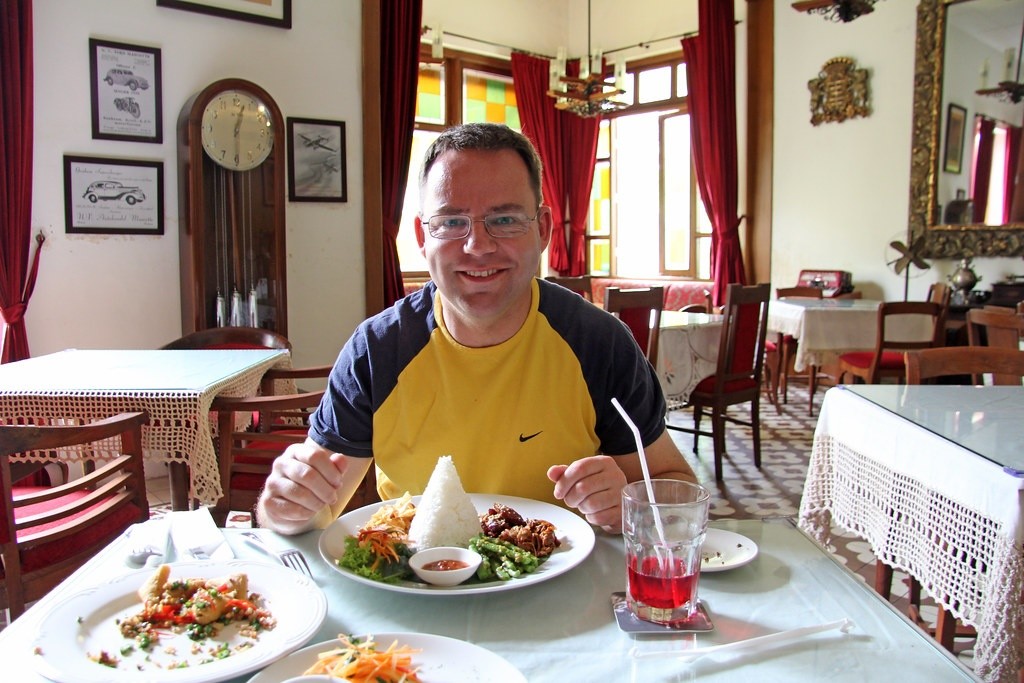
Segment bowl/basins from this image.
[283,675,353,683]
[409,546,483,586]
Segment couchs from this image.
[584,279,716,315]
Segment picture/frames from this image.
[156,1,293,30]
[64,154,165,235]
[89,37,164,144]
[286,115,347,202]
[956,189,966,201]
[943,103,966,174]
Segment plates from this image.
[247,632,529,683]
[318,492,596,595]
[36,560,328,683]
[699,528,760,571]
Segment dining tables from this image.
[592,303,734,456]
[0,349,303,511]
[760,299,939,417]
[797,386,1023,683]
[1,509,992,681]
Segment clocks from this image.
[176,77,287,337]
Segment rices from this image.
[405,456,485,554]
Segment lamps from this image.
[546,0,630,118]
[975,15,1024,104]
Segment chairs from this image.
[152,326,309,511]
[667,282,772,478]
[927,282,951,346]
[604,288,665,373]
[966,308,1024,385]
[775,288,834,418]
[904,346,1024,385]
[544,275,594,303]
[209,363,384,527]
[1,411,151,624]
[838,301,941,385]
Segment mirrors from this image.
[905,1,1024,258]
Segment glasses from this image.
[421,207,542,241]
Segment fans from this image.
[884,230,937,302]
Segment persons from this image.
[255,123,707,540]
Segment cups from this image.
[620,480,712,626]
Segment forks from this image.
[240,532,315,580]
